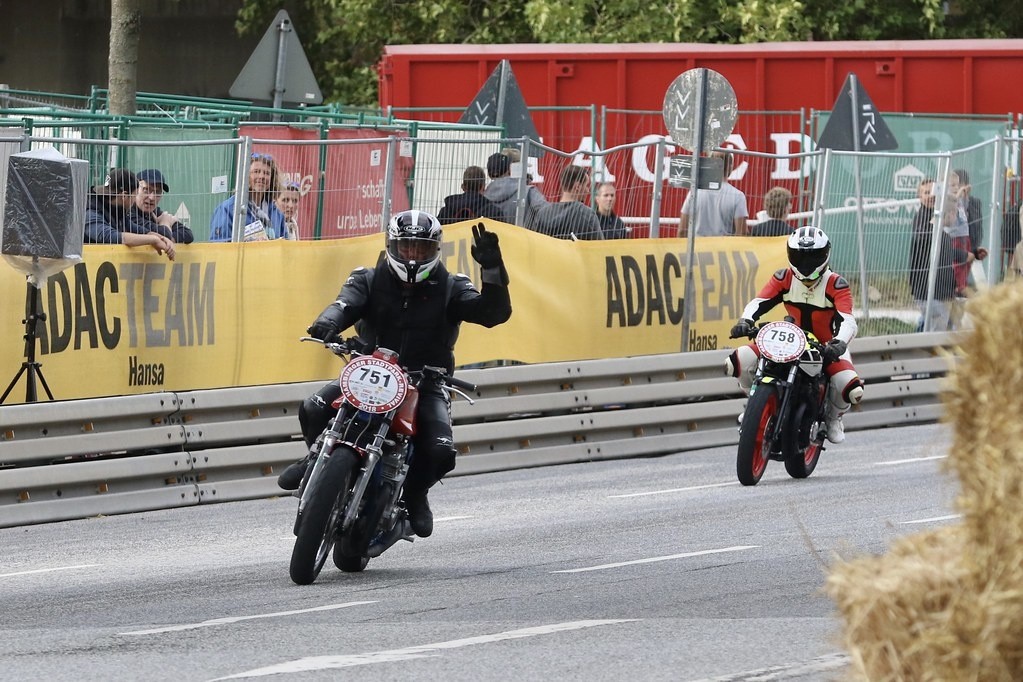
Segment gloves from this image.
[730,322,750,339]
[823,341,840,362]
[305,320,338,348]
[470,223,502,268]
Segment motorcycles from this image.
[289,324,478,586]
[729,316,829,487]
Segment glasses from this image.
[250,152,273,162]
[140,185,164,197]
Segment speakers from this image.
[1,156,89,260]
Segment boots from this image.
[403,461,443,538]
[825,398,852,444]
[736,383,751,424]
[277,437,312,491]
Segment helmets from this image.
[786,225,831,281]
[384,209,442,284]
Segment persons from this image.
[271,182,301,241]
[278,209,514,539]
[1010,203,1023,279]
[483,153,548,227]
[676,149,749,237]
[595,180,627,239]
[909,169,982,332]
[751,186,795,235]
[84,167,176,261]
[126,169,194,244]
[725,226,865,444]
[437,166,506,222]
[530,165,605,240]
[209,152,289,242]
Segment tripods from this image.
[0,255,56,405]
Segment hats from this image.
[93,167,138,196]
[487,154,512,178]
[136,168,170,194]
[280,181,299,190]
